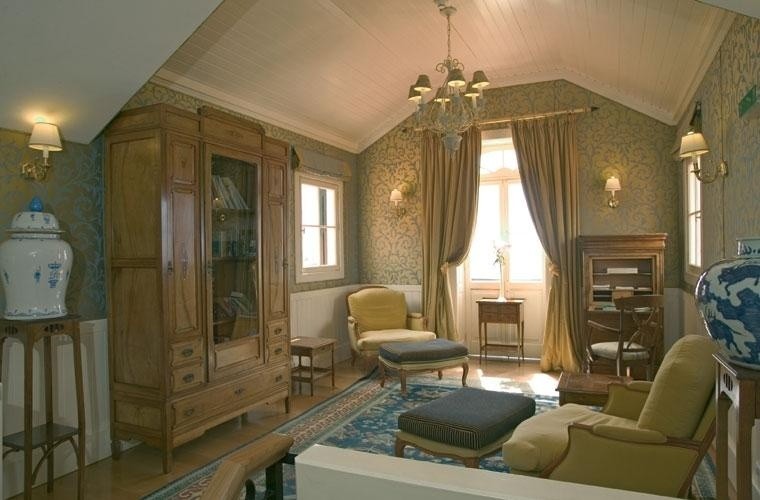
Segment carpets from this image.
[140,364,720,500]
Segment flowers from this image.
[491,232,513,264]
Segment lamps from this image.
[20,122,64,183]
[680,131,728,184]
[604,176,621,208]
[389,188,405,218]
[408,4,490,159]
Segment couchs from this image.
[500,335,718,498]
[394,386,536,468]
[378,338,469,397]
[346,286,444,381]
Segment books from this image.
[594,288,612,291]
[616,286,635,290]
[607,267,638,274]
[594,284,610,287]
[637,286,652,291]
[210,173,258,324]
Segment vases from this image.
[692,237,759,371]
[496,265,507,302]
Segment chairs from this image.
[583,294,665,379]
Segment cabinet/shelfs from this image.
[290,335,337,394]
[0,315,85,499]
[711,352,760,499]
[102,103,292,475]
[477,298,526,367]
[578,232,668,377]
